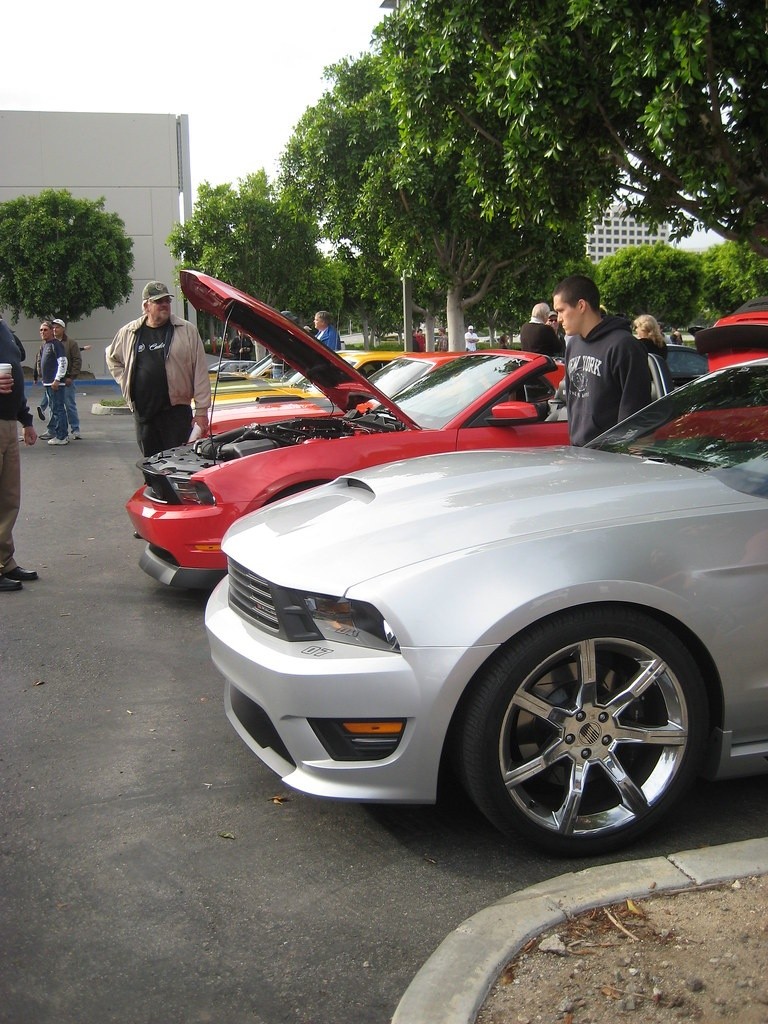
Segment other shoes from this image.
[37,406,45,420]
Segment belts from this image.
[273,366,283,369]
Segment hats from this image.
[43,321,54,330]
[548,311,558,318]
[303,326,312,330]
[468,325,473,329]
[281,311,298,321]
[51,319,65,328]
[142,282,174,301]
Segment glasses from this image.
[40,329,48,331]
[548,319,557,322]
[149,297,172,304]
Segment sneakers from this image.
[48,437,68,445]
[39,432,55,439]
[73,431,82,439]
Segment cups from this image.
[187,421,201,443]
[0,363,13,390]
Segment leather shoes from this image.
[3,566,38,580]
[0,575,22,591]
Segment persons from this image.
[105,281,211,539]
[465,326,478,351]
[412,329,418,352]
[416,329,426,352]
[438,328,448,351]
[0,320,39,591]
[499,334,511,350]
[552,275,652,448]
[11,318,91,445]
[519,303,683,420]
[229,310,341,379]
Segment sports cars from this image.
[123,267,767,859]
